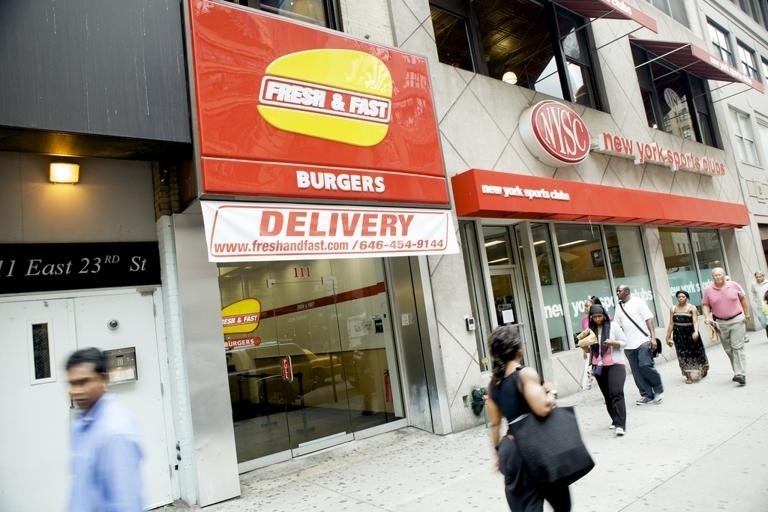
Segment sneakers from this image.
[651,392,663,402]
[615,427,624,435]
[636,396,651,405]
[609,425,615,429]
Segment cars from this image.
[224,337,344,420]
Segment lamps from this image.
[46,156,80,184]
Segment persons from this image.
[483,320,578,512]
[663,290,711,386]
[60,346,150,512]
[748,271,767,332]
[581,294,615,406]
[612,283,666,408]
[585,302,631,439]
[703,265,754,390]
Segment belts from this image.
[714,312,742,320]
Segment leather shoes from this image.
[733,375,745,384]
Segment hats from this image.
[590,304,603,315]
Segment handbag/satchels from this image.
[653,338,661,358]
[510,406,595,498]
